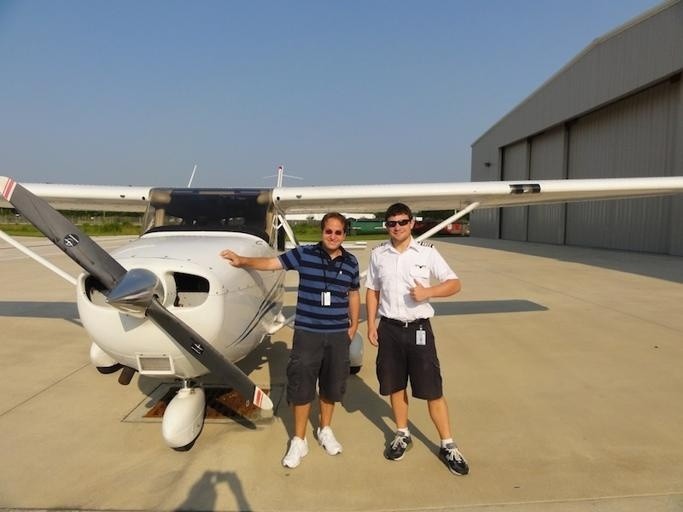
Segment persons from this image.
[219,212,360,469]
[362,201,470,475]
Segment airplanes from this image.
[0,163,683,448]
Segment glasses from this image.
[386,219,410,227]
[325,229,342,236]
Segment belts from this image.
[381,316,427,327]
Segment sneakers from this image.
[316,425,342,456]
[282,437,309,467]
[438,442,469,476]
[386,431,412,462]
